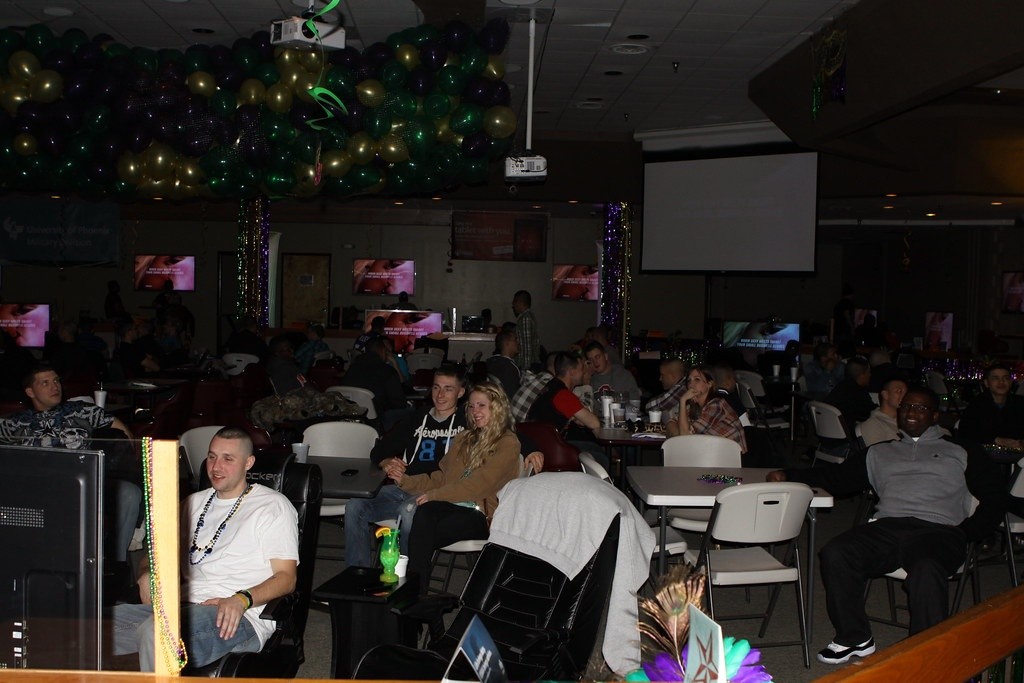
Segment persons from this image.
[119,281,195,378]
[224,319,329,392]
[0,332,39,381]
[763,311,1024,550]
[485,329,519,400]
[44,322,110,383]
[0,361,143,585]
[388,291,417,310]
[344,366,543,593]
[114,426,300,672]
[512,326,643,476]
[646,359,752,466]
[105,281,134,324]
[512,290,540,371]
[767,388,1009,664]
[348,316,408,408]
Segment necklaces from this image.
[142,437,188,677]
[191,483,253,565]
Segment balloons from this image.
[0,17,518,200]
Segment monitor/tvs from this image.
[0,302,51,349]
[0,444,104,671]
[134,254,196,292]
[353,258,416,296]
[722,321,800,352]
[365,309,443,357]
[552,263,598,301]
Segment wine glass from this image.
[379,529,400,582]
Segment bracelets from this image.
[234,590,253,609]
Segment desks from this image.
[625,465,834,670]
[592,428,667,491]
[306,455,387,562]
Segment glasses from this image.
[899,405,928,412]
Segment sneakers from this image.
[817,635,876,664]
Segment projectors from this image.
[270,17,346,51]
[504,156,547,181]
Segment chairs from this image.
[0,347,1024,683]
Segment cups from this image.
[773,365,780,376]
[394,555,408,577]
[292,442,309,463]
[603,397,662,424]
[791,367,798,380]
[94,390,107,409]
